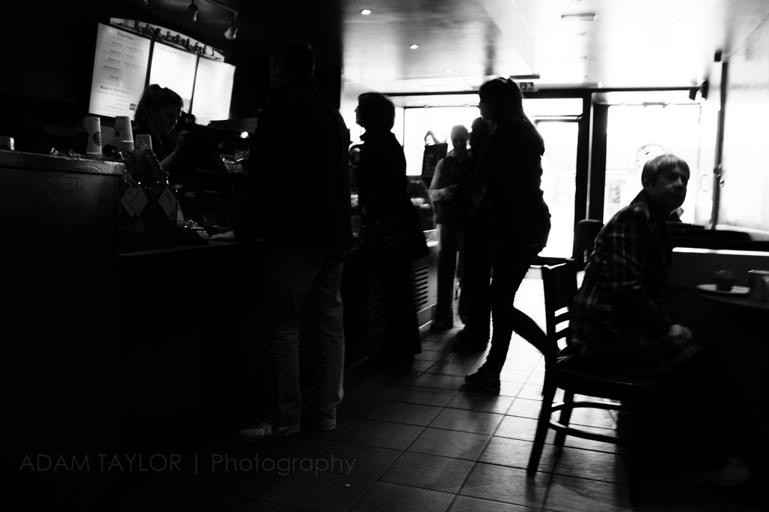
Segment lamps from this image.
[182,0,240,42]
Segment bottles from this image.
[714,269,736,291]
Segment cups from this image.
[83,117,152,158]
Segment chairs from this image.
[526,256,648,488]
[579,220,604,257]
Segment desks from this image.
[696,282,768,316]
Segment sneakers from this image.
[304,404,338,431]
[238,417,303,442]
[430,316,501,395]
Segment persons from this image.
[132,84,184,162]
[566,154,717,512]
[458,76,560,397]
[230,36,352,448]
[426,124,471,335]
[352,91,426,388]
[451,111,495,359]
